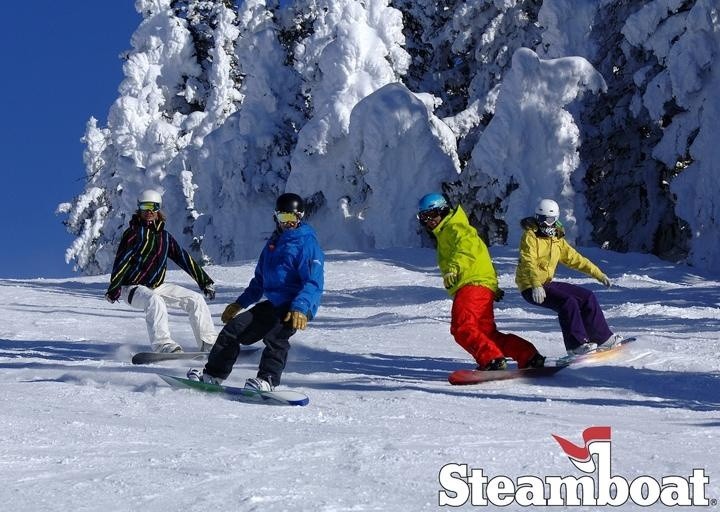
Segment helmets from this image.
[137,190,162,210]
[535,199,560,227]
[276,193,304,222]
[418,193,447,216]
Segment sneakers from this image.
[154,333,623,391]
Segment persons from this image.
[106,190,218,355]
[191,193,324,389]
[516,197,621,355]
[417,194,545,369]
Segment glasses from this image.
[537,215,555,224]
[420,208,441,221]
[139,202,159,211]
[273,210,304,222]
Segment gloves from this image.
[202,282,216,301]
[285,309,308,331]
[106,288,122,304]
[443,271,458,290]
[222,302,241,324]
[530,285,547,306]
[600,275,612,288]
[494,287,505,303]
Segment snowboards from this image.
[447,367,547,386]
[156,371,310,406]
[132,347,260,364]
[543,334,637,367]
